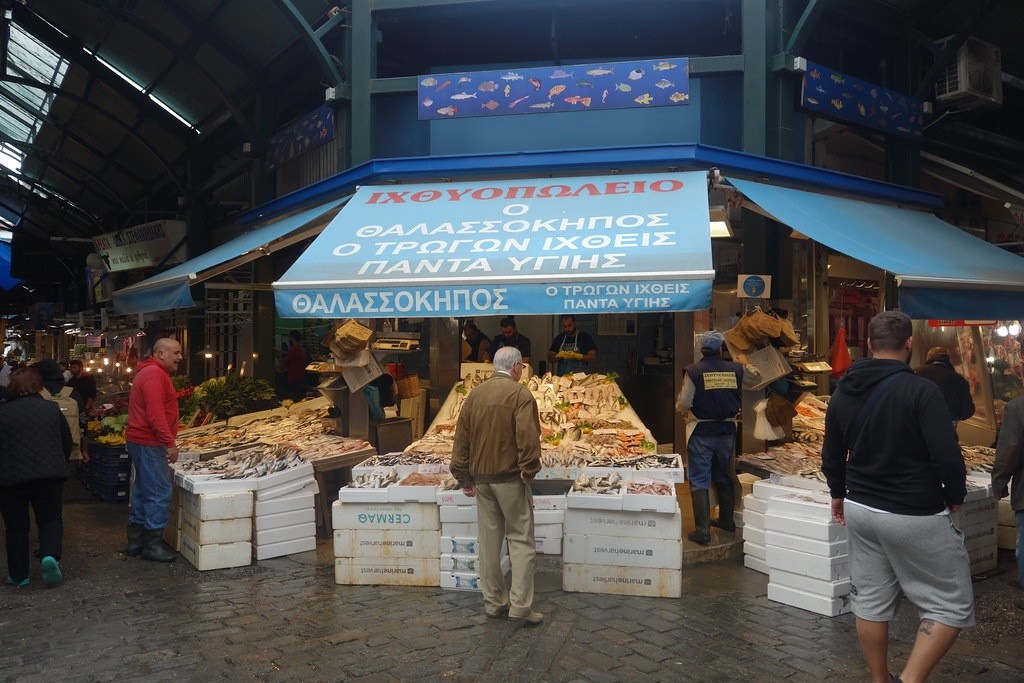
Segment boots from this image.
[711,483,736,532]
[688,489,712,543]
[125,524,143,556]
[140,529,176,562]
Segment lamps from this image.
[87,253,103,269]
[710,205,734,238]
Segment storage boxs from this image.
[332,441,510,591]
[531,454,684,599]
[461,363,534,379]
[793,392,831,422]
[401,389,427,440]
[716,472,1015,618]
[76,433,132,503]
[129,396,330,571]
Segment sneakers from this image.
[508,609,544,624]
[485,603,510,618]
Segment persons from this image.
[450,347,545,625]
[123,338,183,560]
[0,356,97,496]
[482,317,531,363]
[911,347,976,428]
[991,394,1024,612]
[676,330,743,541]
[281,329,306,402]
[548,315,596,377]
[0,368,73,586]
[462,322,492,363]
[820,311,976,683]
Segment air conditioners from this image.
[935,35,1003,110]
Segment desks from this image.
[312,446,377,538]
[322,415,414,455]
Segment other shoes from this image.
[6,576,30,586]
[40,556,62,587]
[1014,599,1024,614]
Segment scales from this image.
[304,355,334,371]
[370,331,422,350]
[784,328,832,391]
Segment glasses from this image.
[513,361,527,371]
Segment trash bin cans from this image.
[369,416,415,455]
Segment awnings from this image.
[270,170,716,319]
[726,172,1024,321]
[113,191,352,315]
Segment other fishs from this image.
[419,61,689,116]
[347,372,678,501]
[734,422,997,489]
[174,409,369,481]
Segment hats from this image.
[700,331,725,352]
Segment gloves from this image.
[555,350,583,361]
